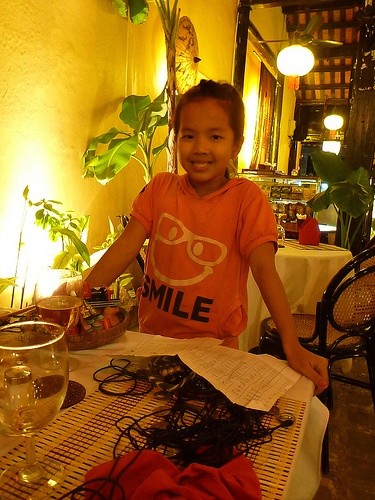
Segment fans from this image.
[257,14,344,48]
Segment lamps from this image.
[277,30,316,77]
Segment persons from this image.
[83,79,328,396]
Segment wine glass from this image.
[0,321,70,500]
[36,295,84,373]
[273,212,287,248]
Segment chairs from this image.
[258,235,375,475]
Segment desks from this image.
[283,223,337,244]
[238,240,353,351]
[0,332,330,500]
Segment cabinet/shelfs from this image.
[237,172,321,235]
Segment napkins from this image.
[84,445,262,500]
[298,217,320,245]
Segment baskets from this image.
[19,303,131,350]
